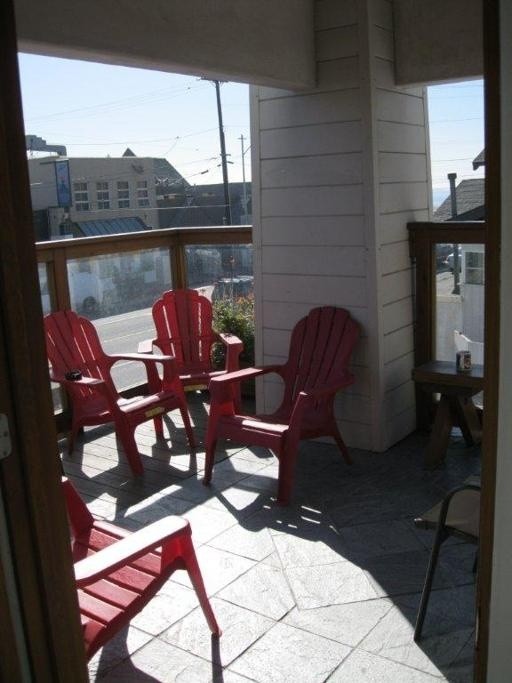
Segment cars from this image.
[447,246,462,272]
[210,275,255,302]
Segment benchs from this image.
[413,360,485,448]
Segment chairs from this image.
[42,308,199,476]
[412,475,482,643]
[139,286,246,442]
[201,304,363,510]
[57,475,225,663]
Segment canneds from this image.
[456,350,472,372]
